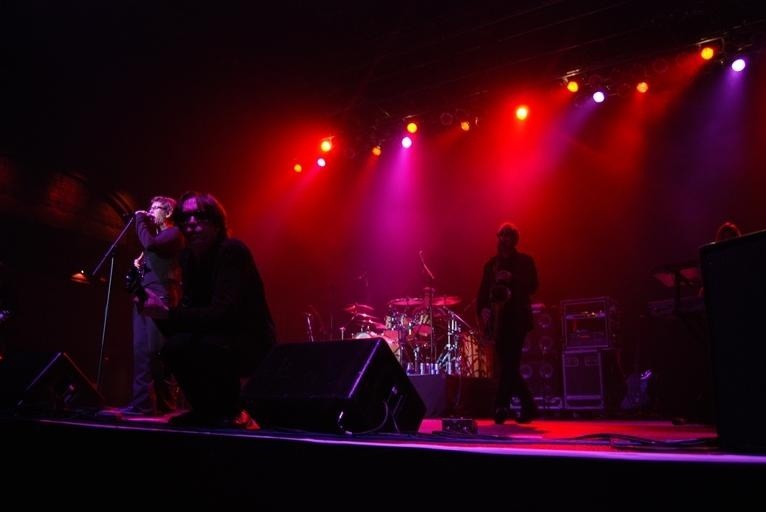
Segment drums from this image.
[459,334,494,375]
[406,325,429,344]
[346,331,404,366]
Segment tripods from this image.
[433,329,462,375]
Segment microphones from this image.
[419,250,425,265]
[128,212,154,222]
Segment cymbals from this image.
[344,305,375,314]
[387,297,420,306]
[427,297,461,306]
[354,315,376,324]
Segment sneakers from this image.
[120,406,153,416]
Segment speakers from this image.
[0,351,105,415]
[239,339,427,434]
[699,231,766,456]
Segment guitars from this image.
[124,265,179,336]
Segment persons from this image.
[712,220,743,243]
[123,195,180,417]
[134,190,273,434]
[474,222,541,425]
